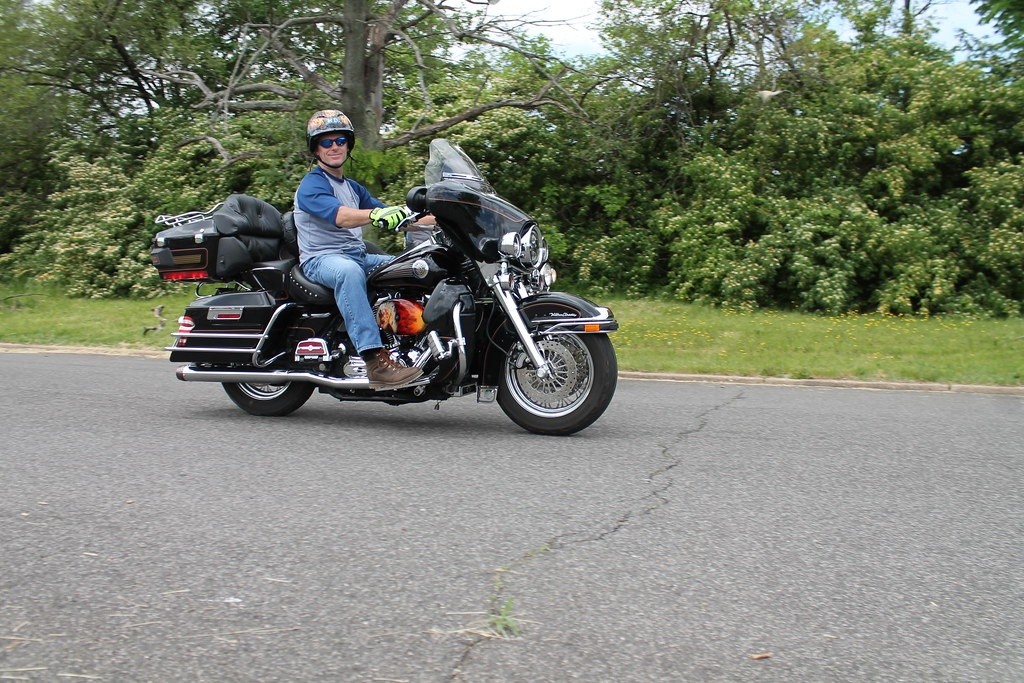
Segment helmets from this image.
[306,110,355,153]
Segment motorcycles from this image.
[148,136,621,437]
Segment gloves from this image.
[369,206,407,230]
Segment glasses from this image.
[318,136,347,148]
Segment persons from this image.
[294,109,437,390]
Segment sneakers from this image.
[363,349,424,389]
